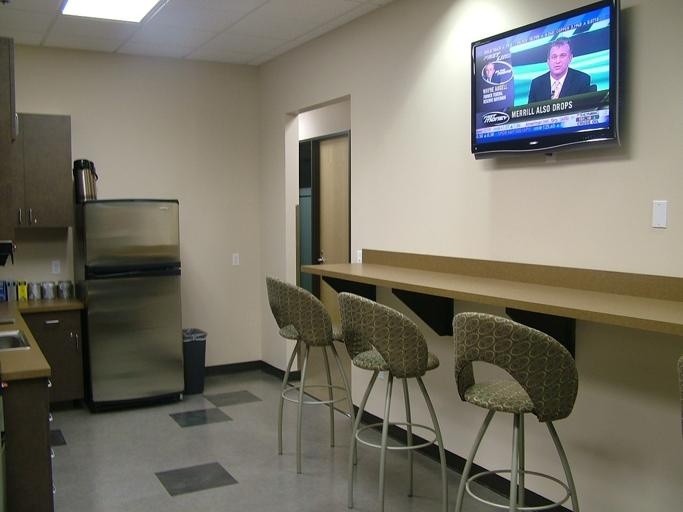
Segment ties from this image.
[553,82,561,100]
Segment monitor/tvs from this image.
[469,0,620,154]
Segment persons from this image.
[528,38,591,104]
[483,63,500,83]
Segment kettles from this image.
[71,159,98,202]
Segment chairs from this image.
[262,275,360,477]
[336,291,452,512]
[448,309,581,511]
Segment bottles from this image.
[58,280,73,299]
[27,280,40,301]
[40,280,56,300]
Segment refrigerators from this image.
[75,199,187,408]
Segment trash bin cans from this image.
[183,328,207,395]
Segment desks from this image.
[299,249,682,363]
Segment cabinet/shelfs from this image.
[7,112,74,229]
[1,34,18,262]
[21,311,84,406]
[0,379,57,512]
